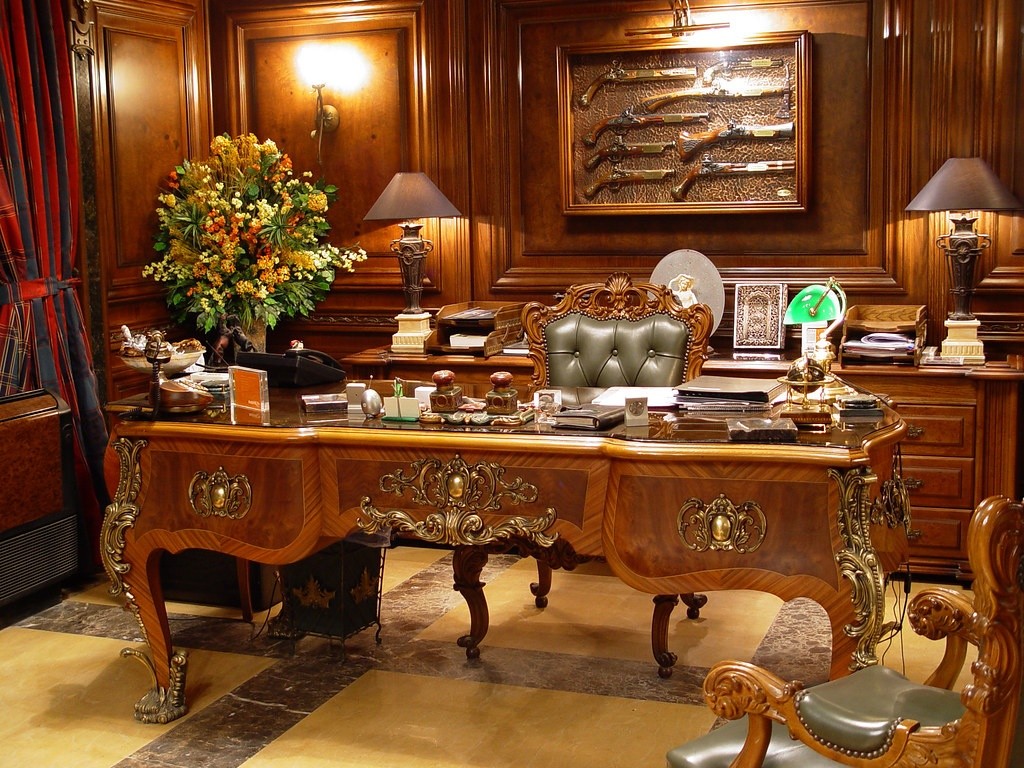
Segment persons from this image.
[666,273,698,308]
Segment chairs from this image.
[664,493,1024,768]
[451,272,714,679]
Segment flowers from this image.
[141,131,369,334]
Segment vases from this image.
[231,310,268,365]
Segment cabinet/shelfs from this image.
[423,300,528,357]
[339,329,1024,588]
[839,304,929,367]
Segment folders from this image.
[672,375,788,402]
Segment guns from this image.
[580,108,711,146]
[641,79,786,112]
[677,119,795,162]
[581,133,677,170]
[669,152,795,200]
[701,56,785,85]
[581,161,675,199]
[579,58,699,108]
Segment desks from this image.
[99,378,909,724]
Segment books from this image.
[549,404,622,430]
[724,417,797,443]
[833,394,884,424]
[502,343,529,355]
[449,333,488,347]
[671,375,787,412]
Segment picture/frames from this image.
[555,27,813,217]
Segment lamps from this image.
[904,159,1024,364]
[363,171,463,354]
[293,40,371,161]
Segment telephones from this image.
[237,348,346,388]
[117,329,215,421]
